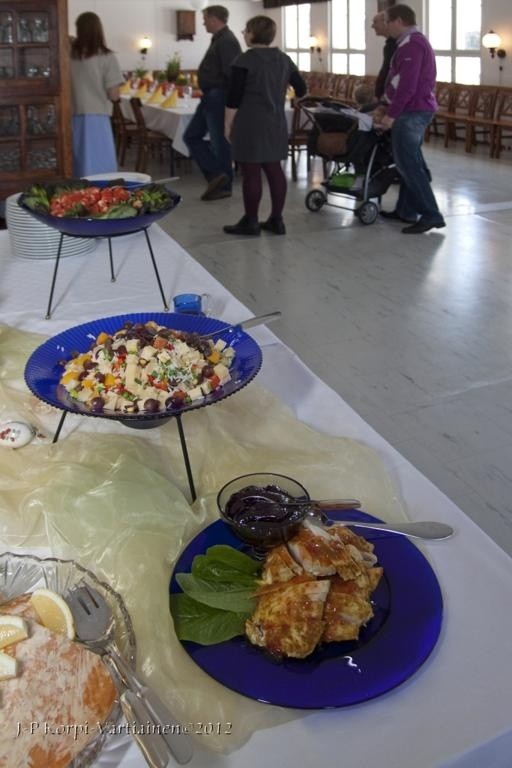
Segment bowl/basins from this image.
[217,472,312,547]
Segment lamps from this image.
[307,35,324,54]
[479,30,506,58]
[139,35,151,55]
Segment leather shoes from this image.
[379,209,417,224]
[401,212,447,235]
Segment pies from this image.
[245,521,383,661]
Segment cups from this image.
[171,294,203,312]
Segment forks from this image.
[60,586,193,768]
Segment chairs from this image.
[102,60,509,229]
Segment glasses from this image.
[383,19,391,27]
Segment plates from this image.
[24,312,263,422]
[168,501,444,709]
[0,552,134,767]
[4,172,181,257]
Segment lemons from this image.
[0,615,29,681]
[31,588,76,641]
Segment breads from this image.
[0,593,116,767]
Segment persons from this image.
[371,11,433,181]
[353,84,380,110]
[183,5,242,201]
[223,15,306,236]
[68,11,126,178]
[376,4,446,234]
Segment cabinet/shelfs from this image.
[0,0,73,182]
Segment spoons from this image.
[302,507,454,544]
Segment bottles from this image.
[0,14,48,43]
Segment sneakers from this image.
[223,215,260,237]
[200,173,232,201]
[258,216,286,235]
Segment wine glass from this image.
[130,76,195,109]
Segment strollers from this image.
[297,95,431,226]
